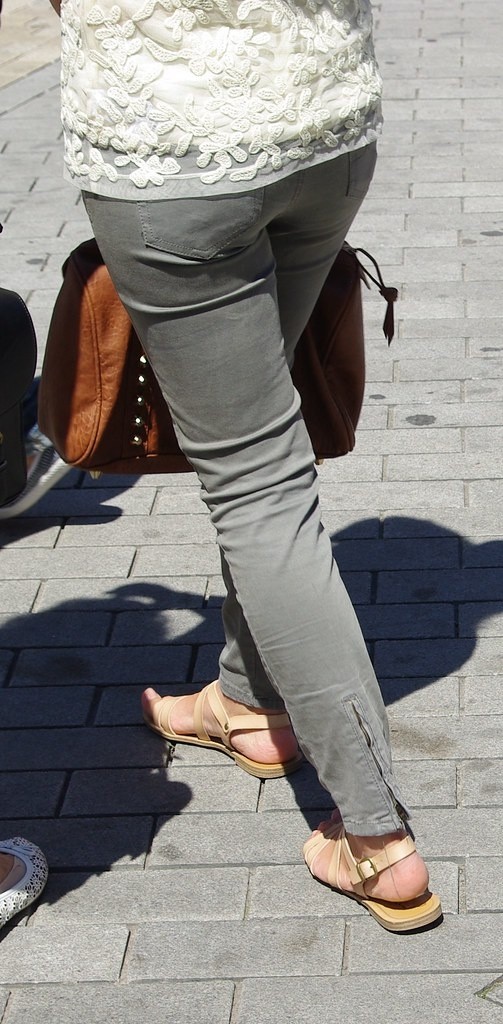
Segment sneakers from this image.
[0,424,74,519]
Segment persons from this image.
[0,420,75,521]
[46,1,444,931]
[0,836,49,933]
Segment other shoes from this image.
[0,836,48,929]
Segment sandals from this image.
[302,808,442,932]
[140,677,304,779]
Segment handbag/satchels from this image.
[34,236,398,477]
